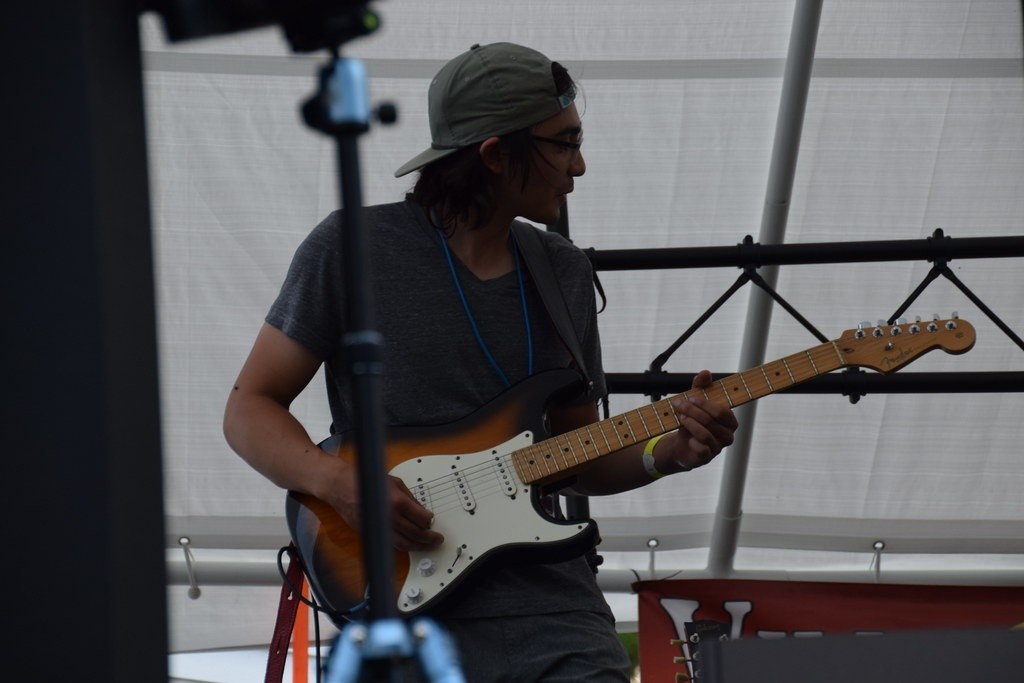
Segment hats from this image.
[395,42,576,178]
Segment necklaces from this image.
[434,216,532,386]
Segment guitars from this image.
[669,621,731,683]
[283,309,979,635]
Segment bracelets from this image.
[643,430,675,479]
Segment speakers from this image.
[701,626,1024,683]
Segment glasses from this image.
[517,127,584,168]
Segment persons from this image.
[222,43,737,683]
